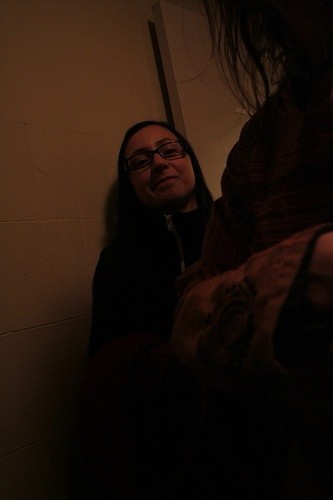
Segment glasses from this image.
[122,140,185,171]
